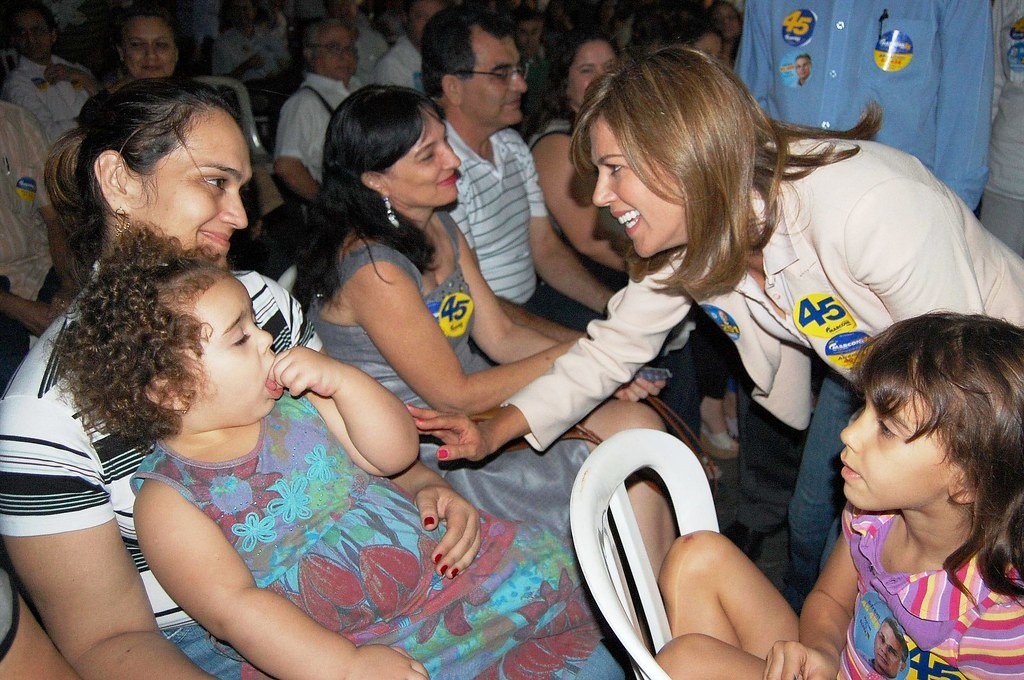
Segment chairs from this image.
[188,75,268,174]
[569,428,721,680]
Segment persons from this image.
[722,0,995,561]
[294,84,677,582]
[0,0,742,482]
[980,0,1024,251]
[48,226,608,680]
[854,617,908,680]
[792,54,812,89]
[0,78,627,680]
[408,44,1024,617]
[1008,47,1019,64]
[656,312,1024,680]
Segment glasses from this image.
[453,61,525,79]
[307,40,357,55]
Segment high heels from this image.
[700,422,739,460]
